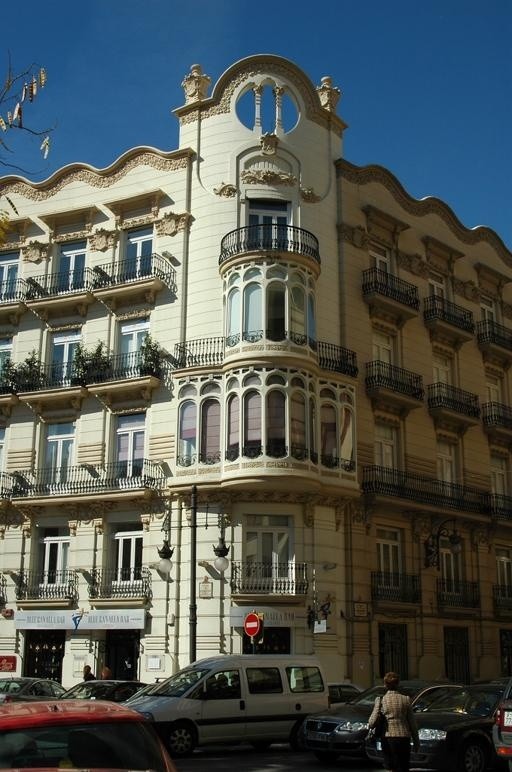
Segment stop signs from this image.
[243,613,261,637]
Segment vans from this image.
[119,655,330,759]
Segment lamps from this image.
[425,518,462,572]
[213,510,231,571]
[157,511,175,573]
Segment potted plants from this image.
[0,333,161,395]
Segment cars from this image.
[297,683,512,771]
[0,698,177,772]
[0,677,65,701]
[58,680,151,705]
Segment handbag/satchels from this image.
[376,713,389,741]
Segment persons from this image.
[101,667,112,680]
[83,665,96,681]
[367,672,421,772]
